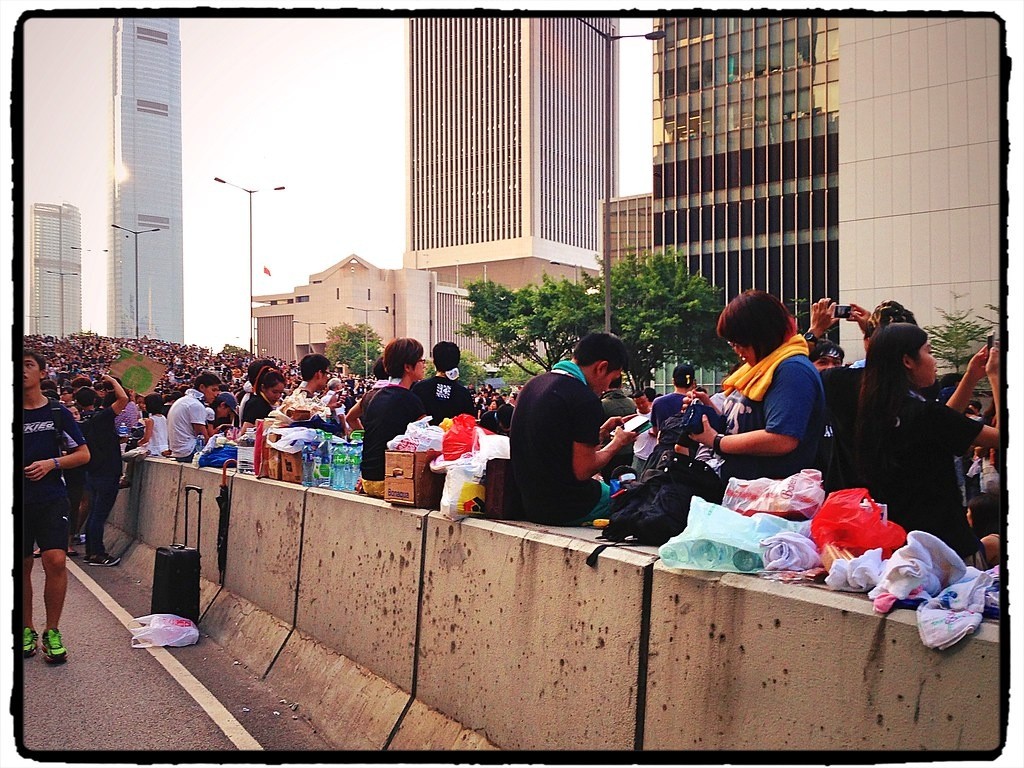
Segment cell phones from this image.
[987,331,995,360]
[336,388,345,403]
[833,305,853,319]
[609,415,649,437]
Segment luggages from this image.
[151,485,203,626]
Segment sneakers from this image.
[89,552,120,565]
[83,555,89,562]
[41,629,68,662]
[23,628,38,656]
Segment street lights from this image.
[604,31,669,331]
[111,224,161,339]
[47,270,79,339]
[215,177,286,362]
[292,320,327,354]
[27,315,49,334]
[71,246,109,331]
[346,306,387,379]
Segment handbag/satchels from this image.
[127,614,198,648]
[198,444,238,467]
[254,419,269,477]
[602,399,727,545]
[723,469,825,518]
[980,458,1000,494]
[810,488,907,560]
[125,421,145,452]
[267,426,349,454]
[388,414,521,521]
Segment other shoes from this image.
[67,548,77,555]
[118,480,132,488]
[33,548,41,558]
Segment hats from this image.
[218,392,238,415]
[673,365,695,388]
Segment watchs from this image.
[803,332,818,345]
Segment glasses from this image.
[324,370,331,379]
[418,359,426,364]
[726,339,754,346]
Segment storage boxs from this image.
[278,438,303,486]
[266,428,281,486]
[384,449,447,509]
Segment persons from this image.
[22,290,1000,665]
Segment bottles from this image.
[303,429,363,492]
[237,427,256,475]
[662,539,764,571]
[196,432,205,453]
[119,422,129,453]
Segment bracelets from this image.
[714,434,724,457]
[51,458,59,470]
